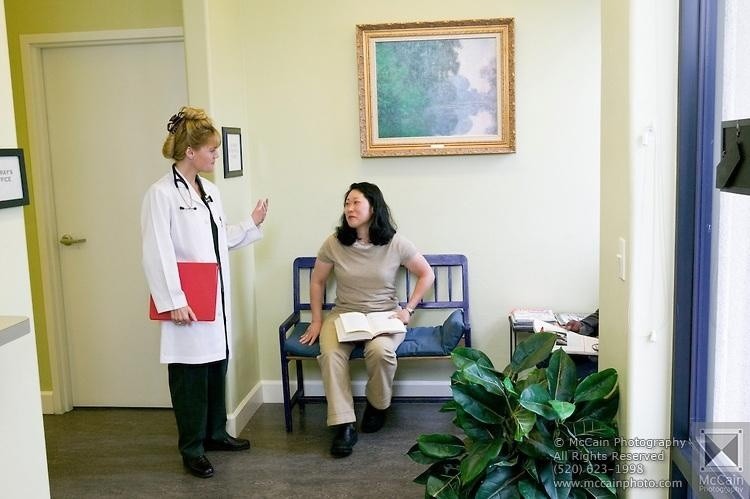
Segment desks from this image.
[508,305,557,363]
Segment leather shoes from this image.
[331,426,358,456]
[182,455,213,477]
[206,435,249,451]
[361,403,387,432]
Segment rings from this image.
[306,335,308,337]
[176,321,181,326]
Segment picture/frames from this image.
[0,148,32,209]
[354,16,516,161]
[221,125,244,179]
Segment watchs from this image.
[405,307,415,316]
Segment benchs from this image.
[278,254,474,434]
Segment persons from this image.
[141,106,270,480]
[300,181,435,459]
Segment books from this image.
[509,307,599,357]
[334,310,408,343]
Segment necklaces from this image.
[351,240,375,250]
